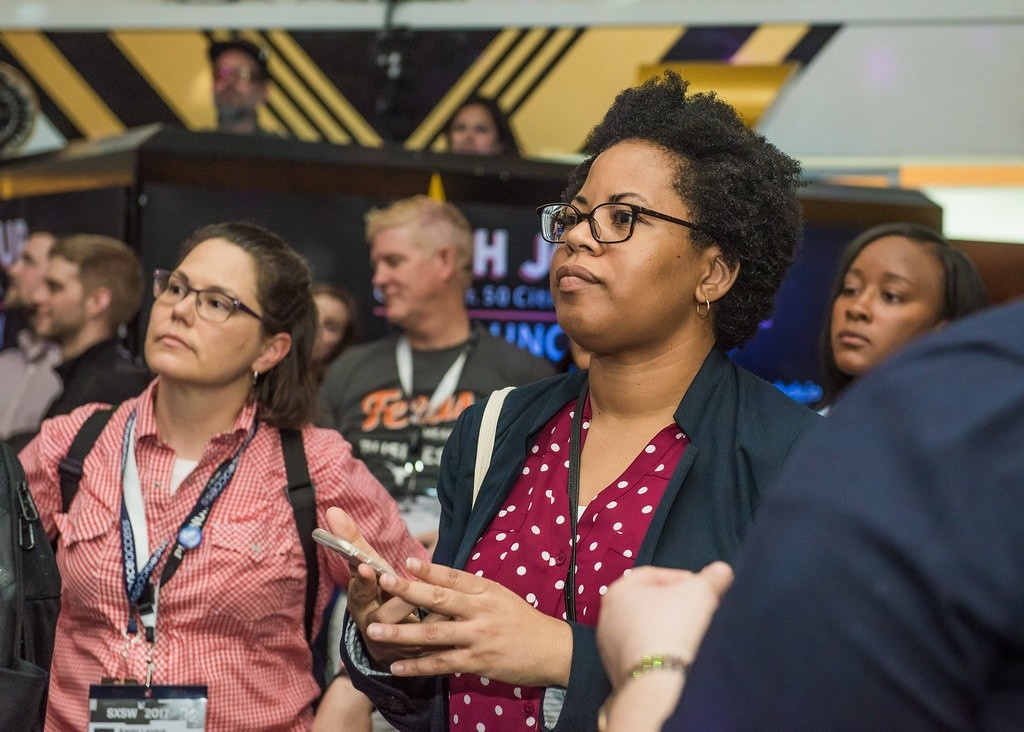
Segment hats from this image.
[210,39,270,84]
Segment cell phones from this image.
[311,529,391,577]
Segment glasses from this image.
[537,202,729,250]
[152,269,268,326]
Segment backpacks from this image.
[0,441,62,732]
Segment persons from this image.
[326,72,827,732]
[0,194,557,732]
[597,224,1024,731]
[446,97,520,158]
[208,38,283,140]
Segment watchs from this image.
[597,654,687,732]
